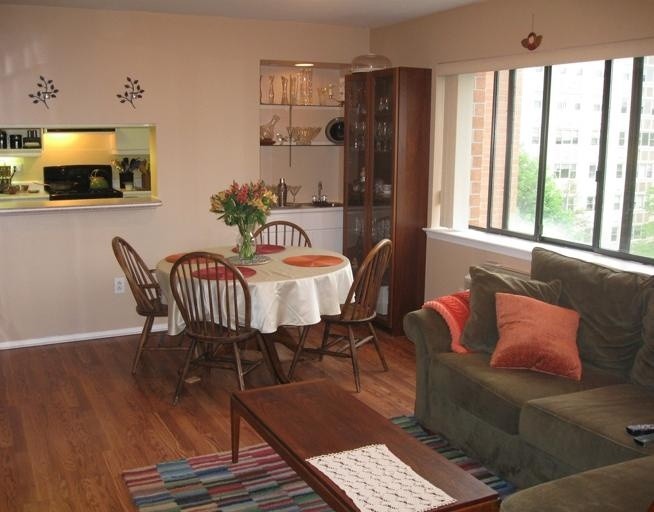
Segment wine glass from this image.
[288,186,302,202]
[351,119,391,151]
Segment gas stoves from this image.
[45,185,123,196]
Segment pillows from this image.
[490,292,582,381]
[458,266,563,358]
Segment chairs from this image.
[239,221,313,365]
[287,237,392,393]
[170,252,280,406]
[111,237,209,375]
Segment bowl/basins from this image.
[285,126,323,146]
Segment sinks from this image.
[311,203,342,208]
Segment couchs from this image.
[402,246,653,508]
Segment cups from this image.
[376,94,392,112]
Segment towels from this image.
[422,289,480,354]
[304,443,457,511]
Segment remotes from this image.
[627,424,654,433]
[633,433,654,448]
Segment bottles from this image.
[277,177,288,208]
[260,113,280,145]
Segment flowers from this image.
[209,179,279,261]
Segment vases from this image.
[235,231,258,262]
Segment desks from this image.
[156,243,351,385]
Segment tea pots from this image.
[87,169,110,190]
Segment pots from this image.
[32,180,81,191]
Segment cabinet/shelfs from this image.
[261,60,347,210]
[344,66,432,332]
[1,120,162,214]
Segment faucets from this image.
[317,181,323,202]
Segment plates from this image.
[324,117,345,145]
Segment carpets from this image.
[122,414,524,512]
